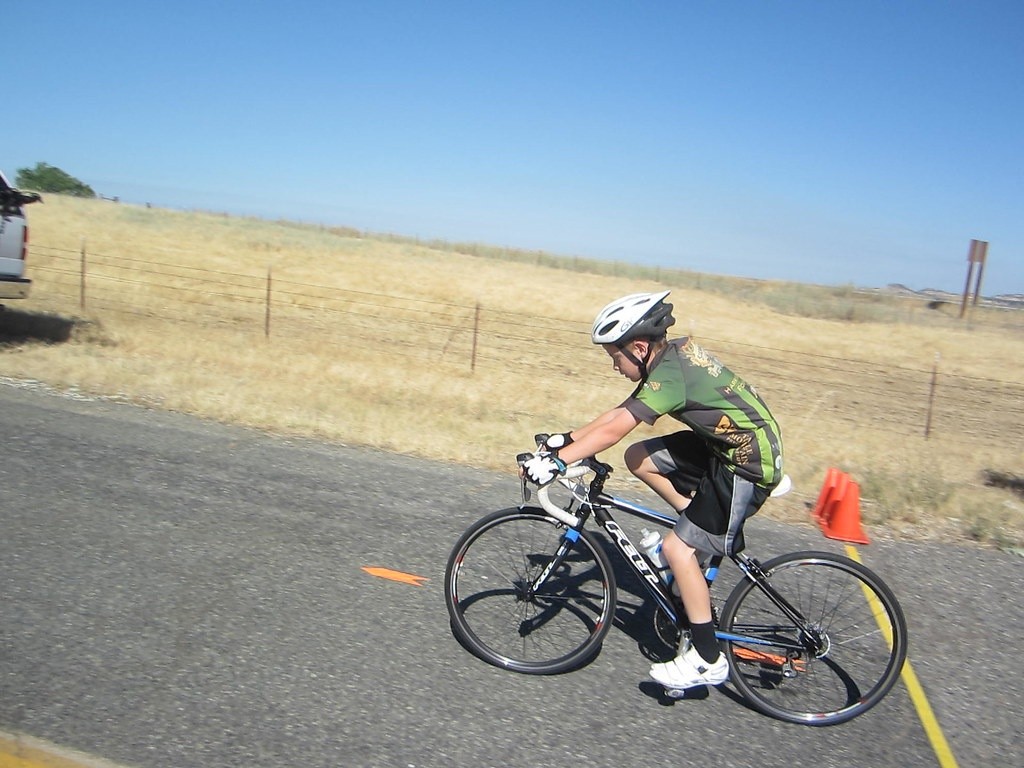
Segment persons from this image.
[519,289,783,689]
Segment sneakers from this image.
[648,645,730,691]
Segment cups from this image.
[639,527,680,597]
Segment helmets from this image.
[590,289,677,345]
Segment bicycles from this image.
[444,433,908,726]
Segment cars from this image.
[0,169,45,299]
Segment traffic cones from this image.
[811,468,850,526]
[823,482,870,545]
